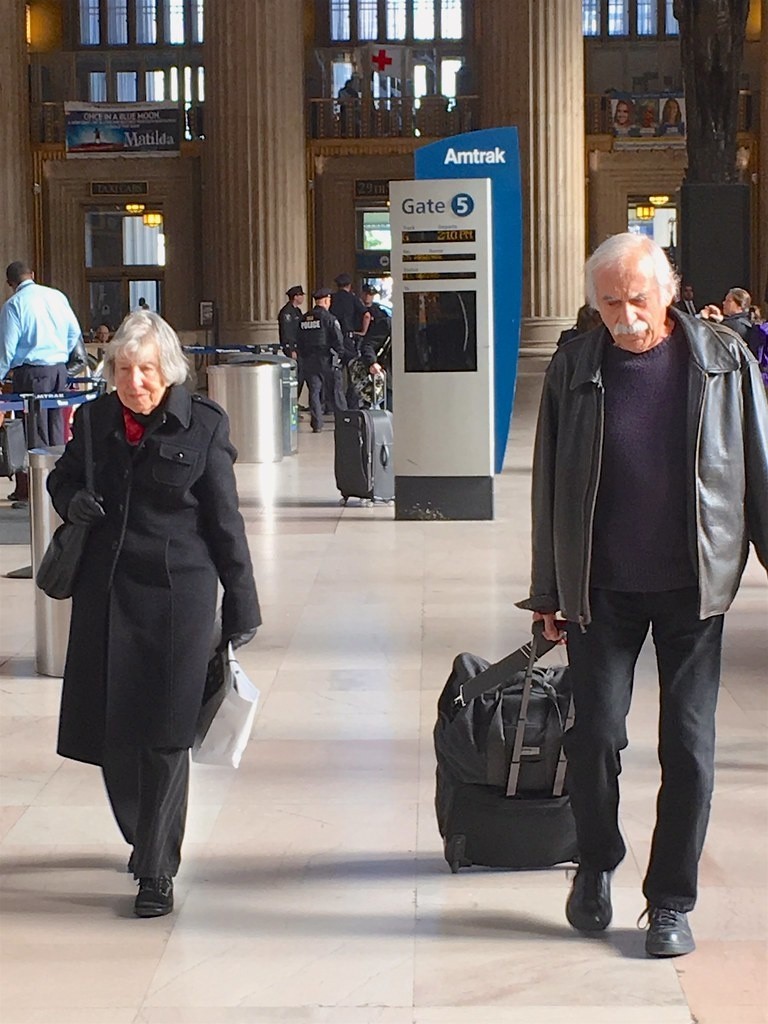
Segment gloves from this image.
[215,624,258,653]
[67,489,106,527]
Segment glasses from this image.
[684,289,693,292]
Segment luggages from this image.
[443,618,578,874]
[0,418,24,481]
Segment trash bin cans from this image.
[27,445,72,678]
[224,353,298,456]
[206,362,283,462]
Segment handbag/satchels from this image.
[35,402,95,601]
[190,600,258,770]
[431,651,575,793]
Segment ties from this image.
[689,301,694,317]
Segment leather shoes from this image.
[566,867,613,931]
[637,904,694,956]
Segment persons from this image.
[338,78,360,138]
[44,309,264,917]
[614,99,636,133]
[661,98,683,124]
[670,284,768,393]
[0,261,90,503]
[577,295,602,336]
[296,286,350,433]
[139,297,149,309]
[515,233,768,960]
[328,273,393,412]
[96,324,110,344]
[275,284,308,410]
[637,99,659,129]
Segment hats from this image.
[312,288,334,300]
[285,285,305,297]
[335,274,353,287]
[361,283,377,295]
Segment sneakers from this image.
[133,874,174,916]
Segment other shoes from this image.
[296,402,334,432]
[8,491,17,501]
[12,499,28,509]
[333,370,396,506]
